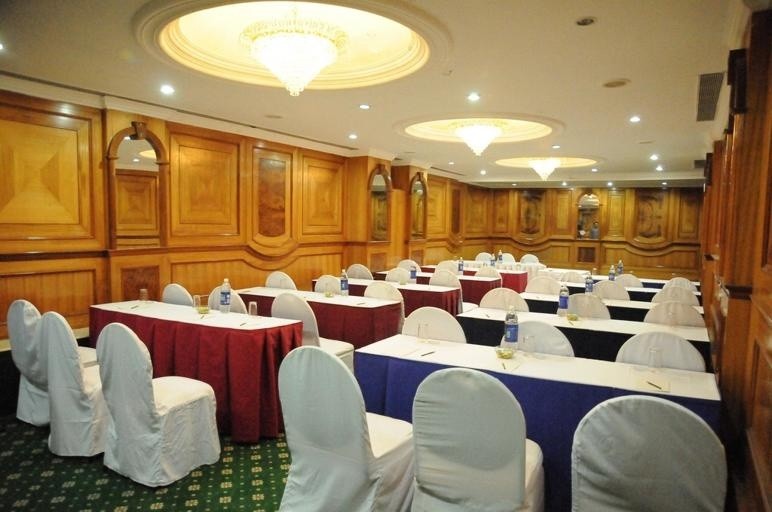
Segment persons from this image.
[590,222,599,239]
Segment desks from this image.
[354,335,720,465]
[89,299,302,445]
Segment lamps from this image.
[241,10,349,97]
[449,119,508,156]
[528,157,560,180]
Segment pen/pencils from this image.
[648,381,662,389]
[200,315,204,319]
[239,322,246,326]
[502,363,506,369]
[421,352,434,356]
[131,305,138,309]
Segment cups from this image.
[192,295,201,311]
[418,321,430,343]
[521,335,539,358]
[248,301,258,318]
[139,288,149,305]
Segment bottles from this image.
[221,278,231,316]
[617,259,623,274]
[498,249,502,264]
[583,272,594,295]
[609,264,616,282]
[555,282,570,318]
[409,261,417,285]
[339,268,349,297]
[490,253,496,266]
[457,257,464,275]
[502,306,519,356]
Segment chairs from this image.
[411,369,545,511]
[94,323,221,489]
[34,311,105,457]
[8,299,97,428]
[570,396,726,512]
[156,252,723,454]
[276,345,415,511]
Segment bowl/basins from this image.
[325,292,334,297]
[198,306,209,314]
[495,347,515,359]
[566,313,577,320]
